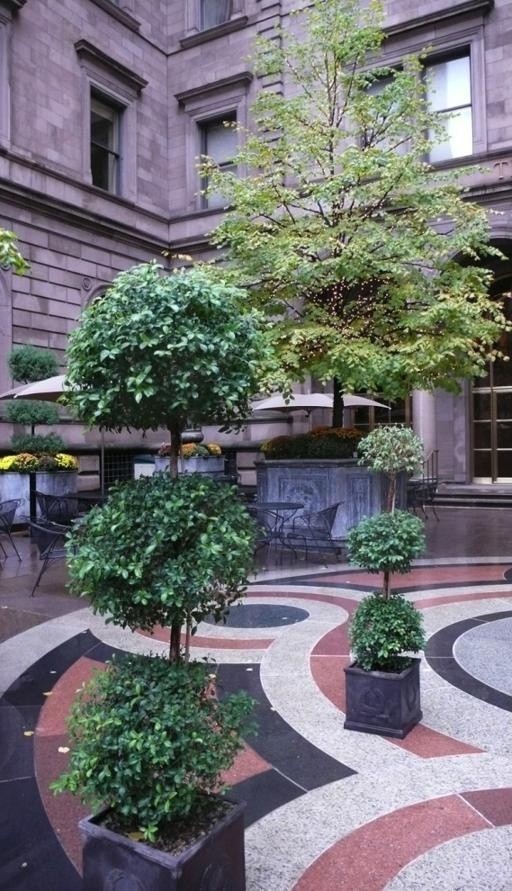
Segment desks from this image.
[236,485,258,502]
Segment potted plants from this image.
[47,649,259,890]
[343,592,423,741]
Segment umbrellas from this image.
[228,389,397,435]
[0,380,45,405]
[12,372,117,495]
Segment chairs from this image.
[409,450,442,522]
[0,489,115,596]
[246,502,342,572]
